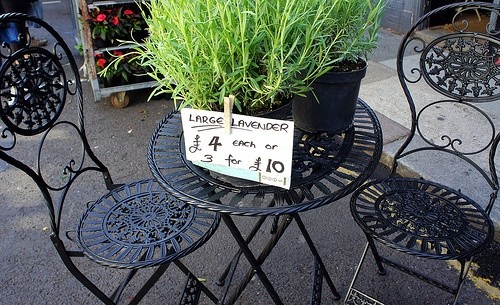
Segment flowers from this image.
[74,4,144,81]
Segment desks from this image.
[148,100,382,305]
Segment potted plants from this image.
[96,0,391,135]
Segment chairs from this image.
[0,12,222,305]
[348,2,500,305]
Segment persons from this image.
[0,0,47,66]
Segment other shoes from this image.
[28,39,49,48]
[14,53,31,65]
